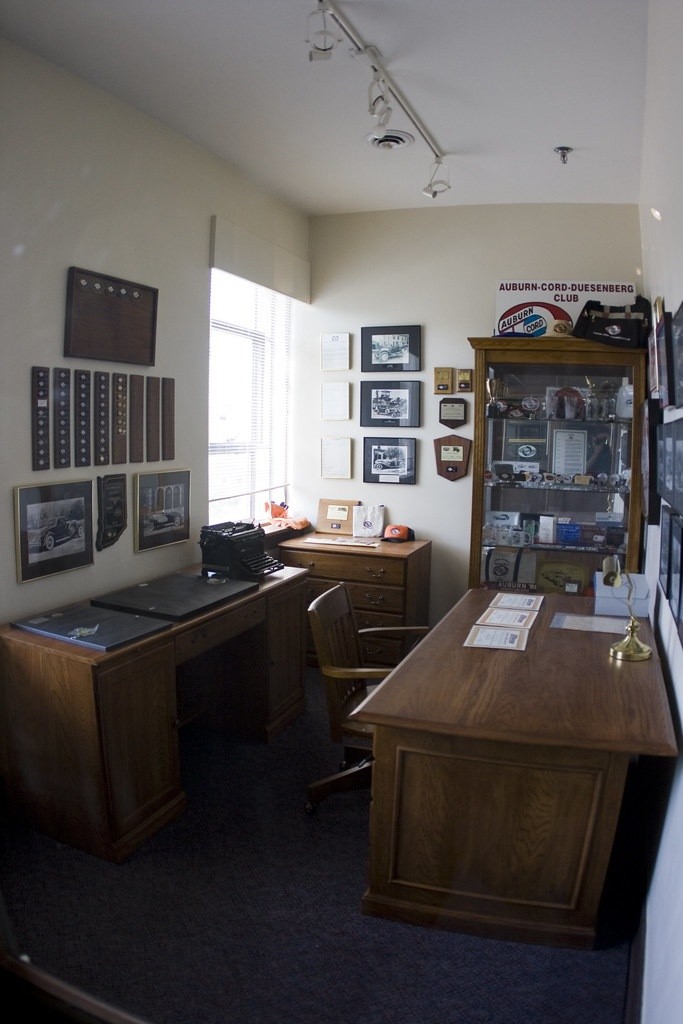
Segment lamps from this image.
[422,156,441,198]
[306,5,340,60]
[601,554,653,661]
[368,76,392,139]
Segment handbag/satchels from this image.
[573,296,652,350]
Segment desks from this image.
[0,563,309,860]
[348,587,678,951]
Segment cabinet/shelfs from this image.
[468,337,648,592]
[276,532,432,666]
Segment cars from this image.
[373,457,405,471]
[28,516,84,550]
[144,511,184,531]
[374,342,409,363]
[373,390,407,419]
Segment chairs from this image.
[306,582,430,798]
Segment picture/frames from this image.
[14,480,94,583]
[132,470,191,553]
[363,437,417,484]
[360,325,421,372]
[64,266,159,367]
[360,381,420,427]
